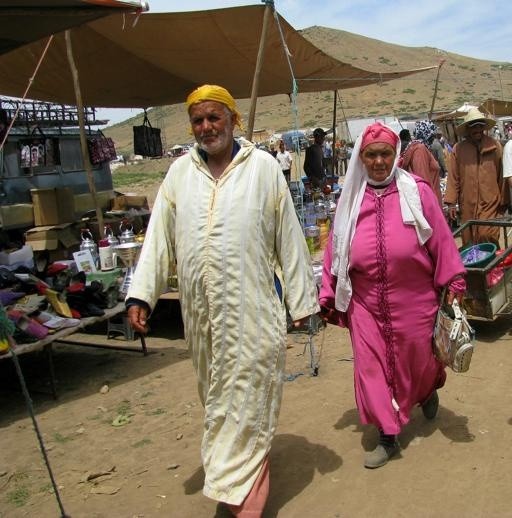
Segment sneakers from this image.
[417,391,439,420]
[364,428,401,469]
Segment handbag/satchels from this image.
[433,286,474,373]
[87,128,117,164]
[133,111,162,157]
[18,126,61,168]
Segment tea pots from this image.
[117,220,136,243]
[80,229,98,269]
[104,227,119,246]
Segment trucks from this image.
[0,115,115,231]
[281,131,307,151]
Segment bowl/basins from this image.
[459,242,497,266]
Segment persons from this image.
[125,83,322,518]
[318,123,469,469]
[258,117,512,221]
[443,106,508,250]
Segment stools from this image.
[106,292,146,343]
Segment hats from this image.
[455,108,496,136]
[313,128,327,137]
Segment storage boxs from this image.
[24,185,150,263]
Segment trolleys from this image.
[448,216,512,331]
